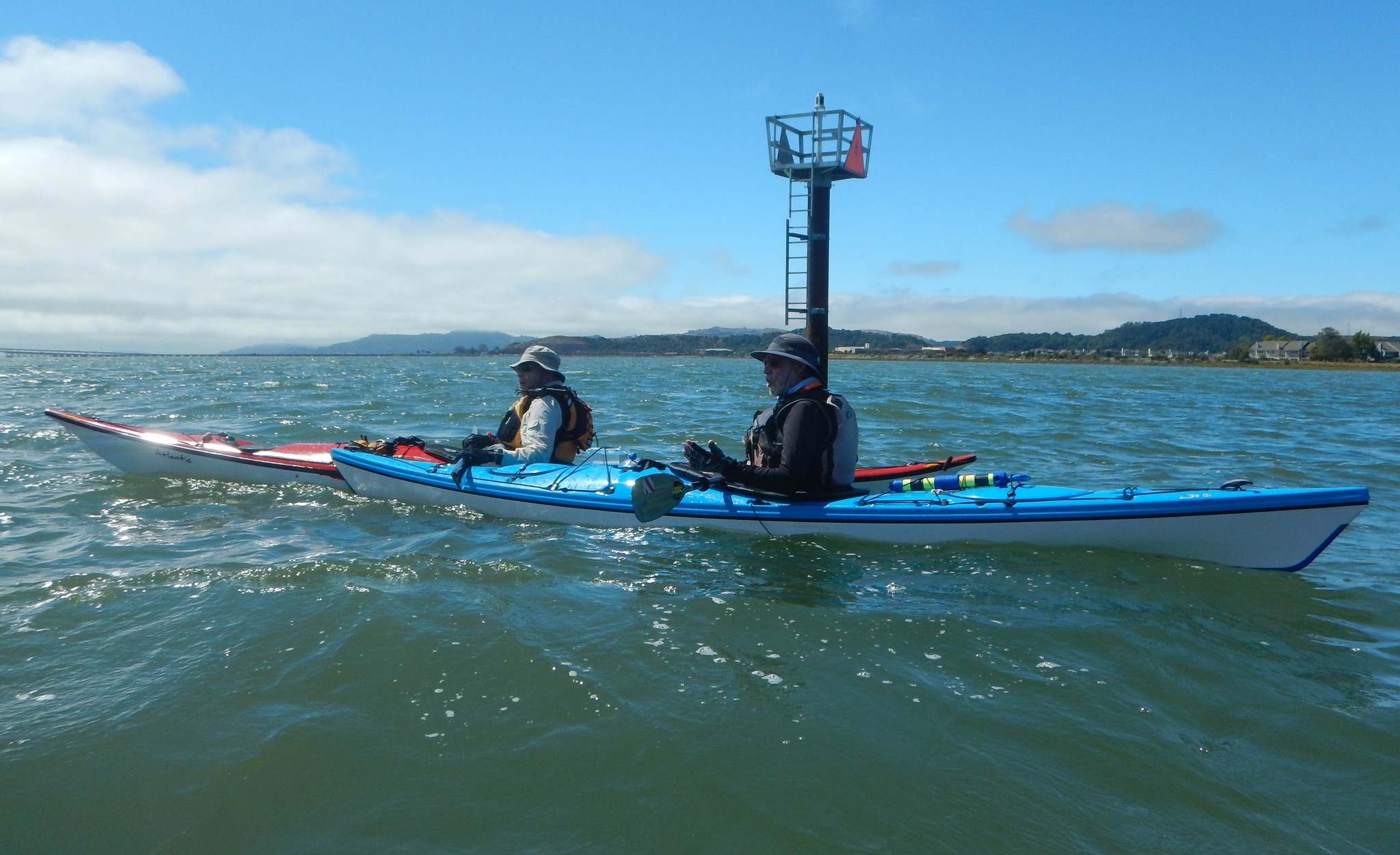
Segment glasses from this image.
[763,356,787,369]
[516,363,540,373]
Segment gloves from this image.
[454,448,490,466]
[462,434,499,449]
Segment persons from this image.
[682,333,858,503]
[455,345,565,467]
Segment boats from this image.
[330,449,1373,573]
[42,405,977,493]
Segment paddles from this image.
[451,432,481,490]
[631,473,728,524]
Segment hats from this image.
[750,333,824,382]
[509,345,566,383]
